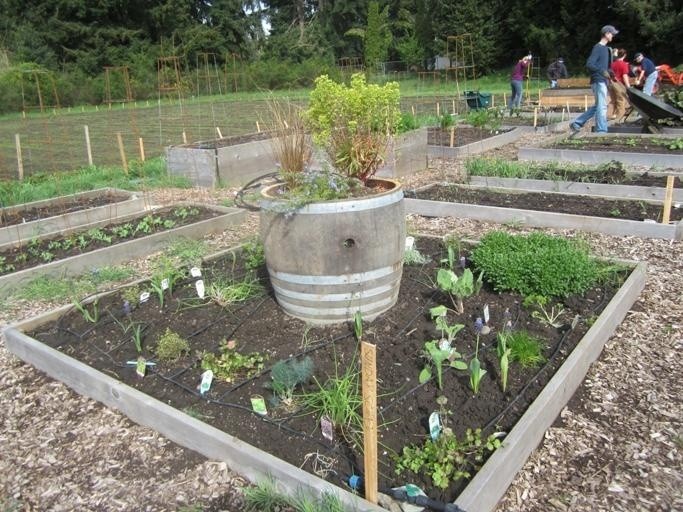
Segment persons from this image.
[569,25,657,133]
[507,55,533,109]
[547,58,568,89]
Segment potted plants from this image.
[258,73,406,325]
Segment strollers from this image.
[463,89,492,114]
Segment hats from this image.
[601,25,619,36]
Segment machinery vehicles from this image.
[631,64,683,93]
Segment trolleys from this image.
[609,74,683,135]
